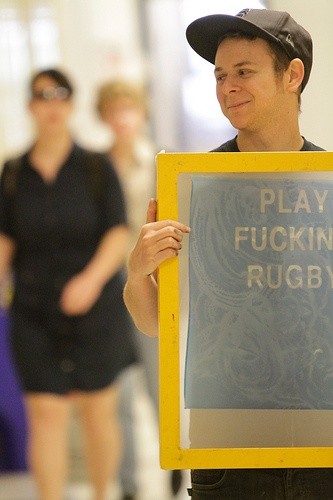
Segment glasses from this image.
[31,86,71,102]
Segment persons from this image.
[0,67,184,500]
[123,9,333,500]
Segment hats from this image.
[186,8,312,92]
